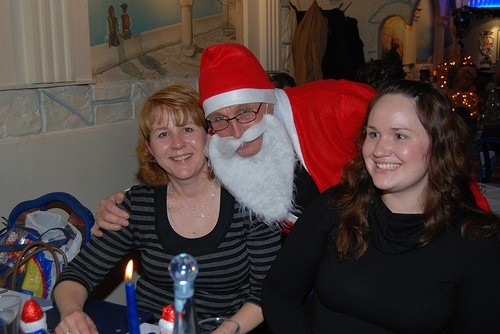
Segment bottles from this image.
[157,304,187,334]
[19,299,48,334]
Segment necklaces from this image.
[168,180,216,233]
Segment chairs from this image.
[6,192,95,250]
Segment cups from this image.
[197,317,240,334]
[477,184,486,196]
[0,294,22,334]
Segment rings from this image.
[66,331,70,334]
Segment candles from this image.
[125,261,142,334]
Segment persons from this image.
[272,73,296,89]
[55,84,282,334]
[262,80,500,334]
[456,40,464,50]
[90,43,491,237]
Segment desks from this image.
[45,297,211,334]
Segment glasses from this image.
[207,100,262,131]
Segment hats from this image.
[198,43,278,120]
